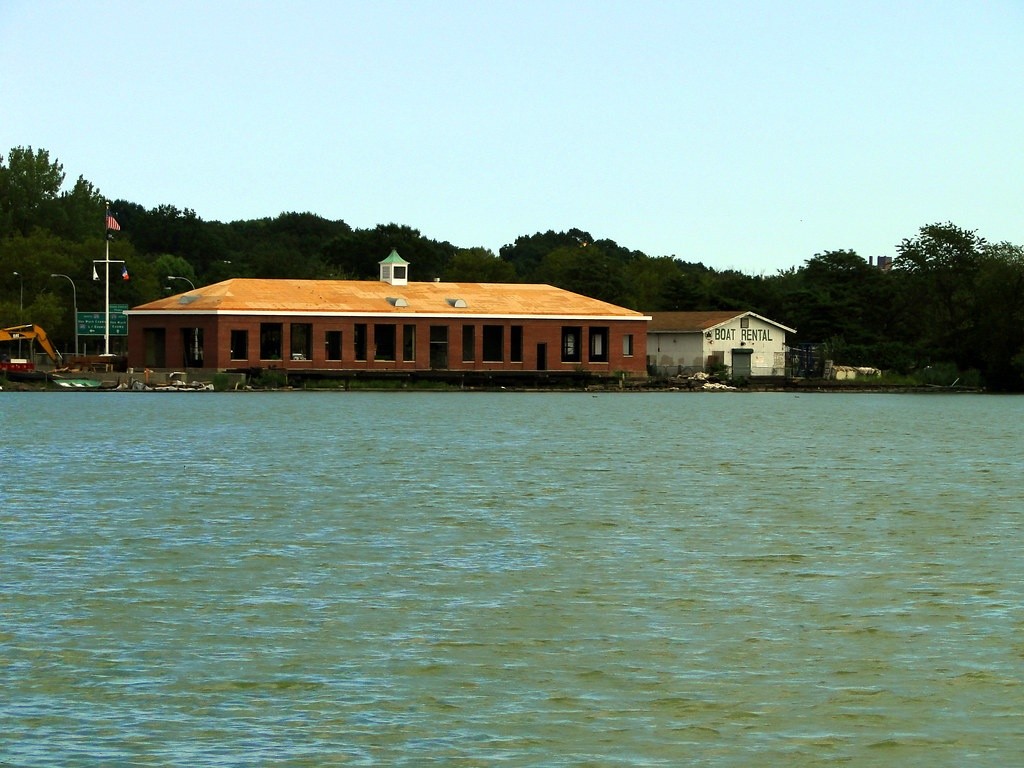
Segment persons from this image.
[261,336,281,359]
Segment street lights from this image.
[51,273,79,356]
[13,272,23,358]
[167,275,195,290]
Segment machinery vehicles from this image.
[0,324,68,372]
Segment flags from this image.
[105,213,121,231]
